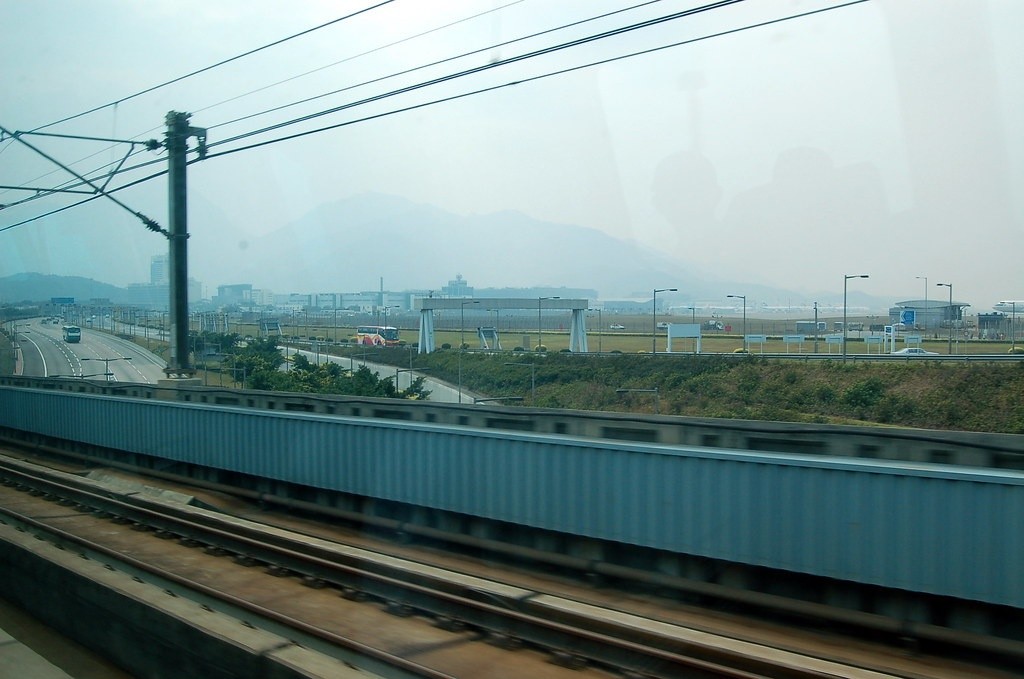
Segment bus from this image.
[356,326,399,346]
[60,326,82,343]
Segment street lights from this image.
[688,308,695,325]
[651,288,678,356]
[936,283,952,355]
[461,301,480,346]
[1000,301,1015,353]
[537,296,561,354]
[915,276,927,338]
[842,274,870,362]
[727,294,746,352]
[80,357,132,382]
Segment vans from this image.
[655,320,672,329]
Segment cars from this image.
[41,316,59,324]
[610,323,625,330]
[890,347,938,355]
